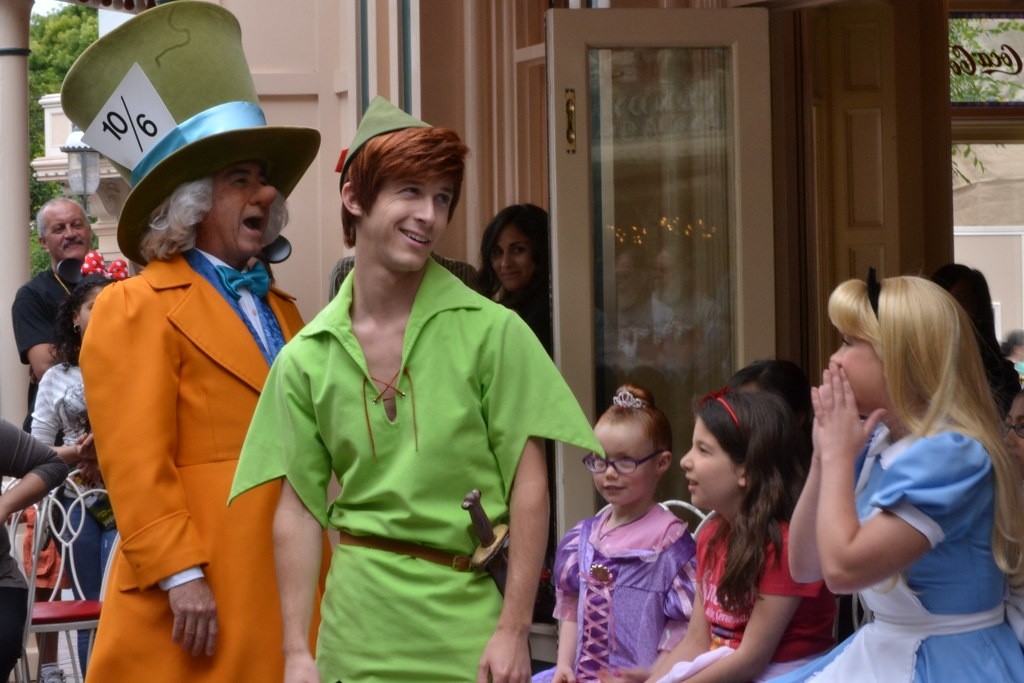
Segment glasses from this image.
[582,450,664,476]
[1000,420,1024,439]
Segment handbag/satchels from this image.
[87,486,117,529]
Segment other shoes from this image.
[40,668,65,683]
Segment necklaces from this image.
[52,272,71,295]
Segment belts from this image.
[339,527,469,573]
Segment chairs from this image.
[0,469,120,683]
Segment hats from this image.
[61,0,320,265]
[340,96,435,190]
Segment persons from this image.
[32,251,133,683]
[60,0,333,683]
[229,96,606,683]
[607,239,732,440]
[482,200,557,362]
[762,266,1024,683]
[639,356,839,683]
[7,505,71,683]
[0,416,72,683]
[9,197,94,449]
[532,382,698,683]
